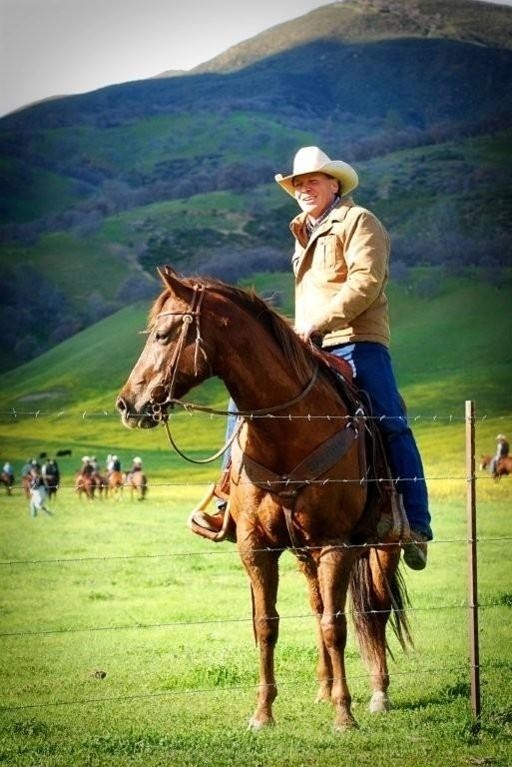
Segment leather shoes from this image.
[403,529,428,569]
[193,506,236,542]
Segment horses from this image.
[116,262,420,738]
[73,470,147,502]
[24,474,58,500]
[0,473,15,495]
[480,454,512,483]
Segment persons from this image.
[2,457,60,519]
[73,453,143,493]
[489,433,510,473]
[190,143,431,572]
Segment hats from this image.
[274,146,359,197]
[82,454,142,464]
